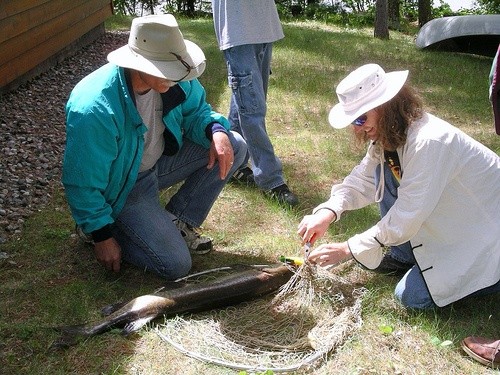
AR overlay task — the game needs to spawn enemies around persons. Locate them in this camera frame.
[212,0,298,210]
[61,14,247,281]
[297,63,500,312]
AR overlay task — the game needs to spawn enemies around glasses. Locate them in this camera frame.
[352,114,368,128]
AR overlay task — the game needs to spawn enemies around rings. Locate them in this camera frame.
[230,162,233,165]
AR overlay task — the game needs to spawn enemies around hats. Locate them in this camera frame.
[107,14,207,81]
[327,63,410,130]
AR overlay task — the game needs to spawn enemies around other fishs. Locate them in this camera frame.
[37,263,295,355]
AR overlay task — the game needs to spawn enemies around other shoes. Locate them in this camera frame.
[167,219,213,256]
[228,167,256,186]
[379,256,407,275]
[76,225,96,247]
[460,336,500,370]
[264,184,301,207]
